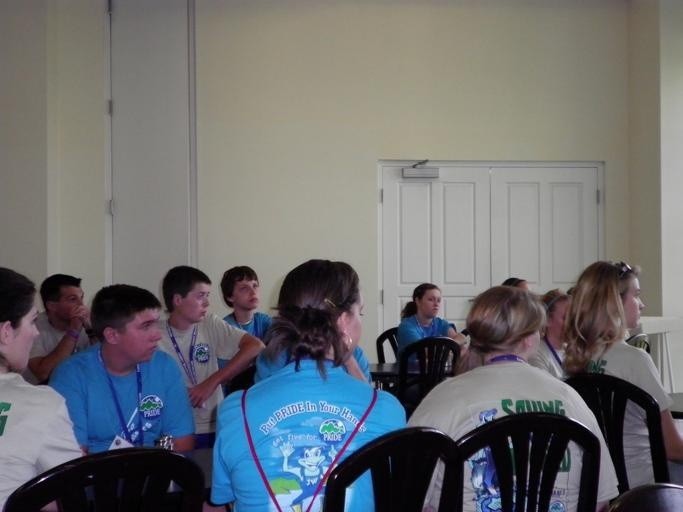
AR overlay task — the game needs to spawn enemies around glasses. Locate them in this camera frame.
[615,261,632,279]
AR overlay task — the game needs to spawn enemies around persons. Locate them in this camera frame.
[397,283,469,409]
[0,265,92,509]
[9,272,104,385]
[405,281,622,511]
[152,262,267,510]
[213,263,278,395]
[502,278,528,288]
[47,283,196,461]
[251,335,373,386]
[205,257,408,511]
[528,259,683,490]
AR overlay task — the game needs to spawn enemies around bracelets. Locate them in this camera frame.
[63,329,81,341]
[85,328,98,338]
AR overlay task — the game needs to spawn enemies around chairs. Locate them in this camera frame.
[320,425,465,511]
[224,366,257,400]
[607,483,680,510]
[1,447,207,510]
[561,371,672,504]
[391,335,462,424]
[446,412,604,509]
[376,326,403,367]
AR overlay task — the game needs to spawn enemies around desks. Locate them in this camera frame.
[367,362,456,421]
